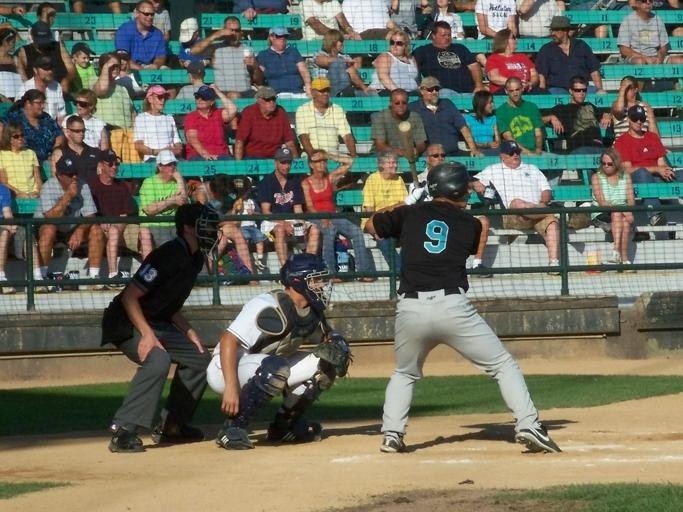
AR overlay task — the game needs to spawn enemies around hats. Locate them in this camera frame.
[542,15,577,28]
[29,20,95,70]
[144,76,329,99]
[627,105,645,119]
[54,148,179,175]
[185,61,204,75]
[498,140,520,154]
[273,147,293,163]
[268,25,290,37]
[178,17,198,42]
[419,76,440,88]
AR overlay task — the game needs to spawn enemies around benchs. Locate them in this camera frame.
[1,10,683,284]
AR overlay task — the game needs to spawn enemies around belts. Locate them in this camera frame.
[403,287,461,299]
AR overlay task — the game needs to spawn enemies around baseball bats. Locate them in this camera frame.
[399,121,419,188]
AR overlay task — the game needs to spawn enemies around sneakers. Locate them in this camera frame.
[622,261,636,273]
[514,421,562,453]
[547,260,560,276]
[0,271,126,294]
[150,423,204,444]
[608,249,620,263]
[108,430,144,452]
[215,427,253,450]
[379,432,406,452]
[470,264,493,278]
[649,213,666,226]
[264,422,321,443]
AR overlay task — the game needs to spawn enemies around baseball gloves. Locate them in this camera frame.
[312,329,351,377]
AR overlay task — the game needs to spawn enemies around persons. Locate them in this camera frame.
[365,161,561,454]
[205,252,351,450]
[0,2,683,294]
[100,203,223,454]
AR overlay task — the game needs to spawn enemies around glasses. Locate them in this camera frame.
[11,99,89,139]
[508,150,520,156]
[630,116,645,123]
[389,39,403,46]
[430,153,445,157]
[572,88,586,92]
[602,161,612,166]
[137,9,154,16]
[424,86,439,92]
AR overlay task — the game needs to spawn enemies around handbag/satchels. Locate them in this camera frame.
[566,212,591,230]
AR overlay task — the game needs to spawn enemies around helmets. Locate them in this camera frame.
[174,202,223,262]
[279,253,333,311]
[426,160,479,199]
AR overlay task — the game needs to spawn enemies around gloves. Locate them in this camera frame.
[403,182,425,206]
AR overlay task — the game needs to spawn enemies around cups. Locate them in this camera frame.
[68,271,79,290]
[52,271,63,293]
[668,222,676,240]
